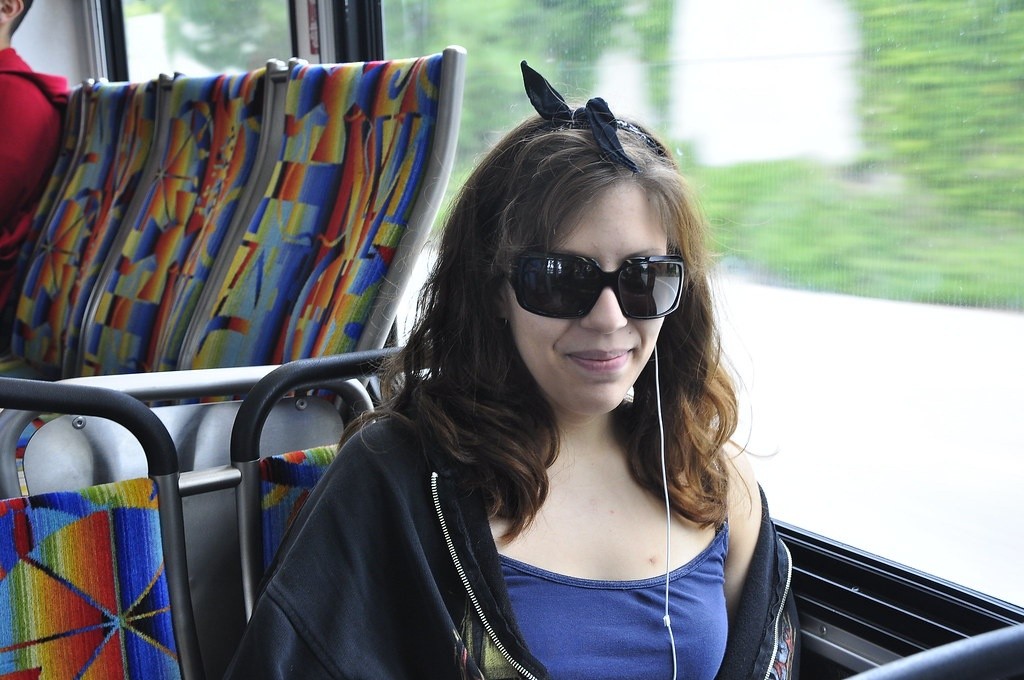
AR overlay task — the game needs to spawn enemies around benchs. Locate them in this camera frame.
[1,45,468,680]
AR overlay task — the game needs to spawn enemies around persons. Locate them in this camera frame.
[223,59,800,680]
[0,0,70,313]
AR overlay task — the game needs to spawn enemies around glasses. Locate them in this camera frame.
[502,250,686,319]
